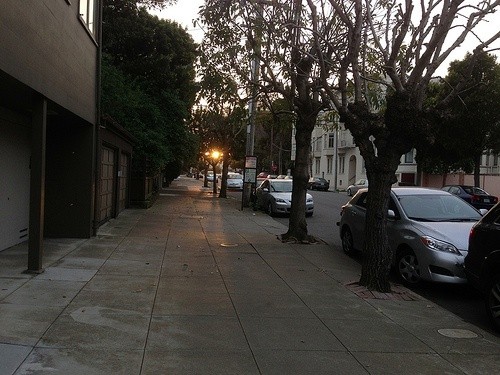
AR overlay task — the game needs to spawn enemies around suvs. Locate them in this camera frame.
[463,202,500,335]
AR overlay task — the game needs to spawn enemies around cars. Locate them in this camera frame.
[346,178,369,197]
[336,185,489,295]
[440,184,499,212]
[194,170,221,182]
[256,179,314,217]
[306,177,329,192]
[256,171,294,188]
[218,172,243,191]
